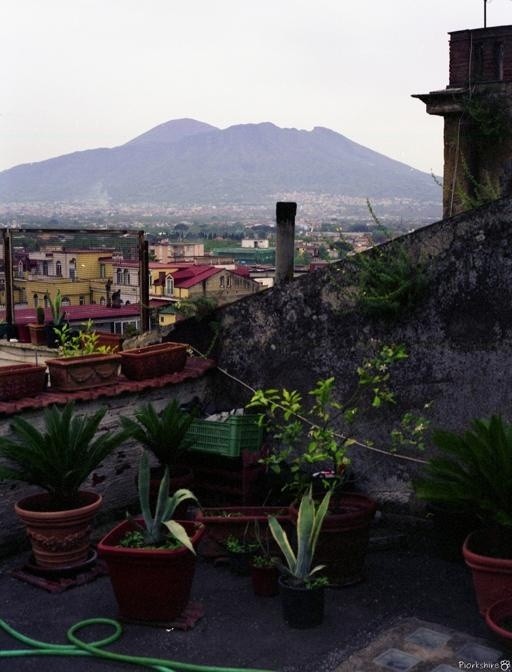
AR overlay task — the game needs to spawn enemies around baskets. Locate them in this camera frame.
[180,413,265,459]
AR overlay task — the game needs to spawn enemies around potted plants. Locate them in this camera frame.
[0,393,328,627]
[417,409,512,616]
[0,289,122,398]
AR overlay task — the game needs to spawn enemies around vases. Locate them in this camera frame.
[484,598,512,650]
[118,341,188,379]
[277,493,375,563]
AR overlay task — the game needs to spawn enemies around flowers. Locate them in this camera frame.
[245,340,430,512]
